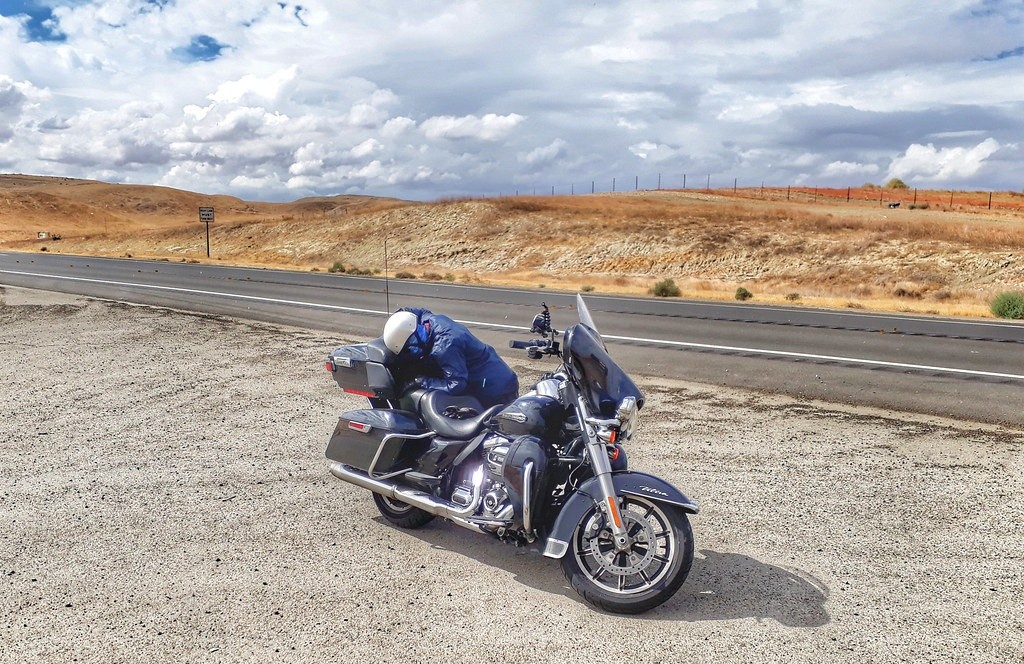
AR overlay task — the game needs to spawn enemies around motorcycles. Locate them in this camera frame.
[320,293,703,613]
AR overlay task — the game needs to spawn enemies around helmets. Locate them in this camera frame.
[384,311,417,355]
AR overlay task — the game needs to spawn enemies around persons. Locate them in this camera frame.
[385,306,520,410]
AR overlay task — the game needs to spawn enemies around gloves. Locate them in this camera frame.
[396,377,416,399]
